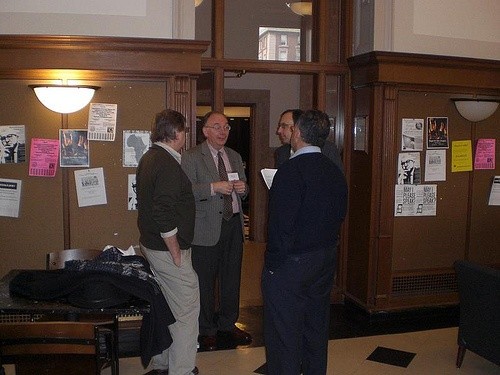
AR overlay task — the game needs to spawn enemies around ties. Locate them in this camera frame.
[217,150,234,224]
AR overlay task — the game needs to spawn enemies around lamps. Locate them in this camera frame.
[448,97,500,122]
[285,2,312,17]
[27,84,101,113]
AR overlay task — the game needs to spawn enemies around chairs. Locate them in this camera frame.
[46,248,115,375]
[0,316,101,375]
[453,258,500,369]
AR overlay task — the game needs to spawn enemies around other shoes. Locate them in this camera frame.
[143,365,199,375]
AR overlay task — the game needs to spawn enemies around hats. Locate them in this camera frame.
[73,279,128,309]
[24,269,63,300]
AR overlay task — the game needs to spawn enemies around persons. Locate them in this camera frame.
[136,109,201,375]
[181,111,252,351]
[275,109,345,176]
[260,109,348,375]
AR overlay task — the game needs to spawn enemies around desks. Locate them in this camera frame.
[0,269,151,375]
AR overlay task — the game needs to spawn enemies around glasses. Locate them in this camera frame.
[205,124,231,132]
[279,123,294,129]
[183,126,190,133]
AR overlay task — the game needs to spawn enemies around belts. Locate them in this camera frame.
[233,211,240,217]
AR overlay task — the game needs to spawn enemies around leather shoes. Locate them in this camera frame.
[217,323,252,345]
[197,329,238,352]
